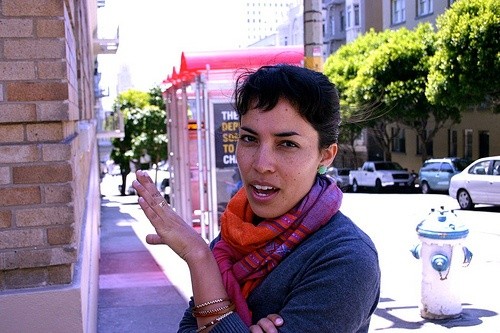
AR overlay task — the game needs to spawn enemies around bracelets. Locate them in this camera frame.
[189,296,237,333]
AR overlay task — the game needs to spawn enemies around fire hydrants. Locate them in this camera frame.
[409,205,473,322]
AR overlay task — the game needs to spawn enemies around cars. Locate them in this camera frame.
[448,156,500,210]
[323,167,353,194]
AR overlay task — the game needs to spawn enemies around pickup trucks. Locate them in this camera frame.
[348,161,412,192]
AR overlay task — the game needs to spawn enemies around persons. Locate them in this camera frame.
[119,148,150,196]
[132,61,382,333]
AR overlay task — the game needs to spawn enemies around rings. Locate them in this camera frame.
[159,200,166,208]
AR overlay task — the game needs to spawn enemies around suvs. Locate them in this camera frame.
[418,157,477,194]
